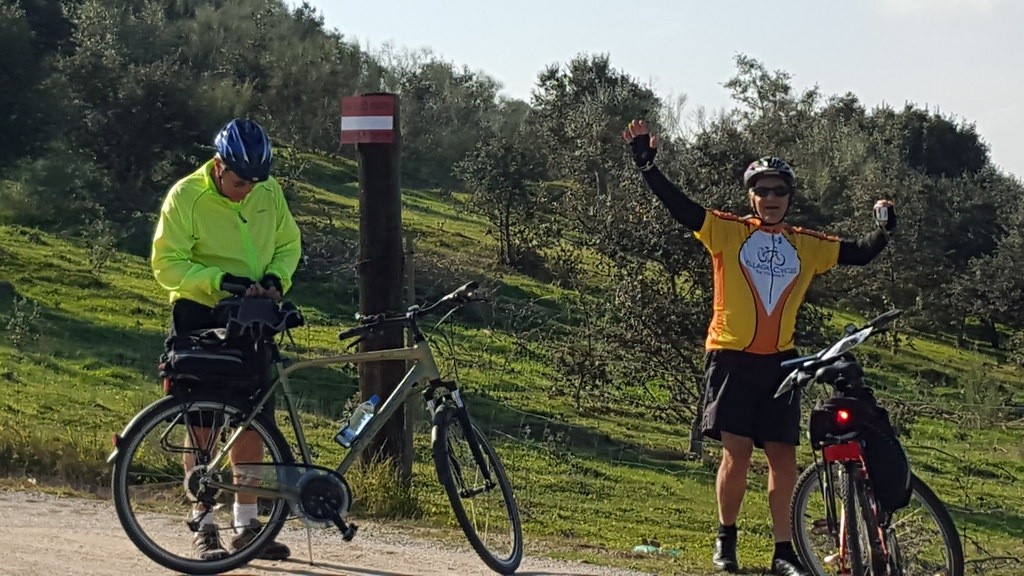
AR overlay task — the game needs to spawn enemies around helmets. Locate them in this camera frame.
[743,156,796,189]
[214,118,273,182]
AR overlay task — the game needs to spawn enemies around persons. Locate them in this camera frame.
[623,119,897,576]
[150,119,301,560]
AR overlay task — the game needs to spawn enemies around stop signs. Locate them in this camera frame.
[340,95,394,144]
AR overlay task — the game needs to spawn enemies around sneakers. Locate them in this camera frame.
[188,524,230,560]
[231,518,291,560]
[770,554,813,576]
[712,533,738,572]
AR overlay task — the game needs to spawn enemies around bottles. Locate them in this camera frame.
[335,395,380,448]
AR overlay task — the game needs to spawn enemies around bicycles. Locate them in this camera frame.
[780,308,964,576]
[107,280,523,576]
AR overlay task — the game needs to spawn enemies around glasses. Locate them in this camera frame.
[751,187,792,197]
